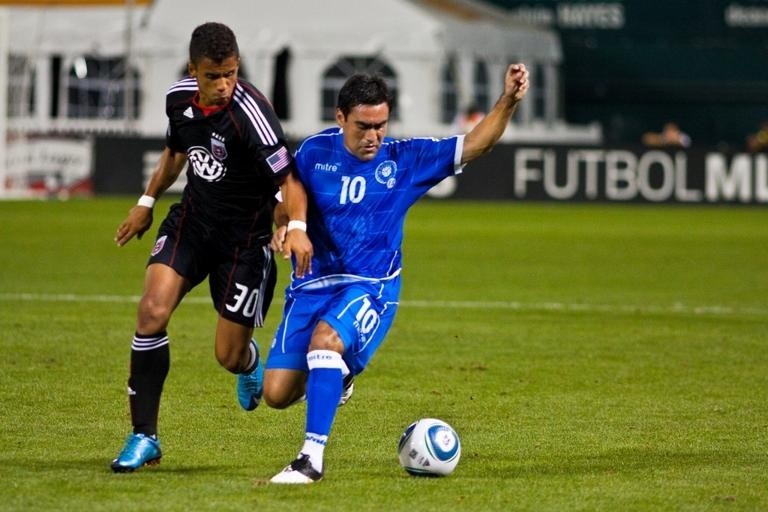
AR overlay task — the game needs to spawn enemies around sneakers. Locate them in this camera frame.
[270,455,324,485]
[236,337,264,411]
[110,432,162,473]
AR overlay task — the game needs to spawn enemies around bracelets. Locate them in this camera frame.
[136,194,157,208]
[287,220,307,232]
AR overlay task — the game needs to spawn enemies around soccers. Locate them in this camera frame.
[396,419,461,476]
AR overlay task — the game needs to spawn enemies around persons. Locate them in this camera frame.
[263,62,529,483]
[110,22,314,473]
